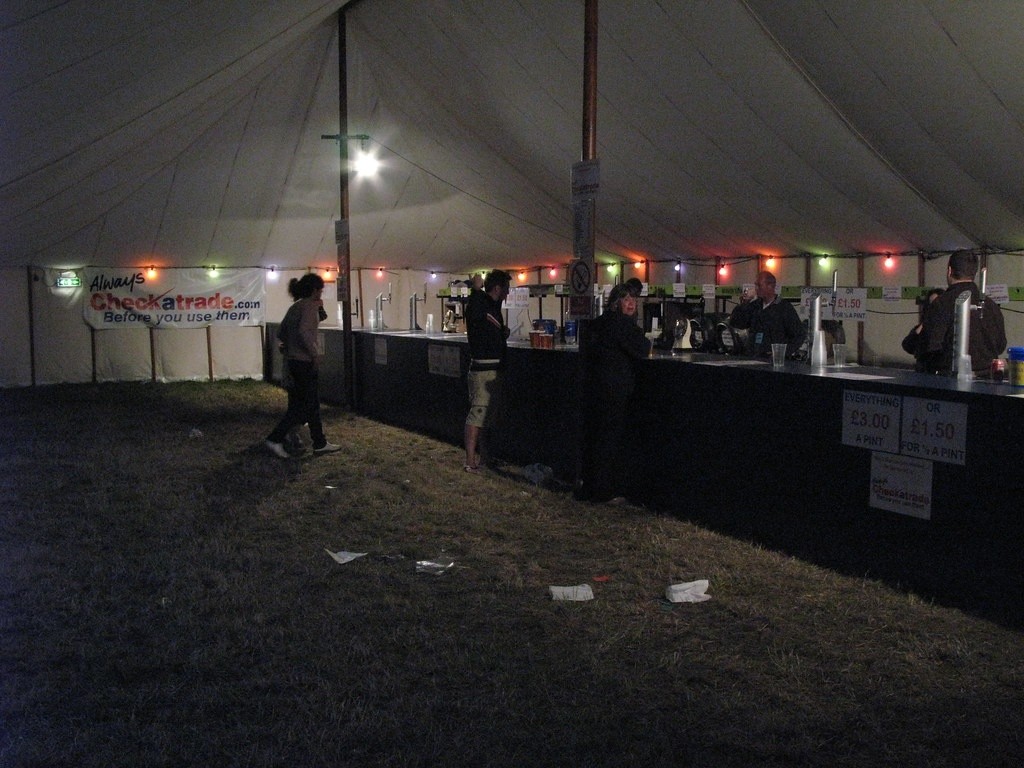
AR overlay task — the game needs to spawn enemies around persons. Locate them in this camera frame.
[730,272,806,356]
[584,278,651,505]
[264,273,341,459]
[463,269,512,473]
[902,250,1008,378]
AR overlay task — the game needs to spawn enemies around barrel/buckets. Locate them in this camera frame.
[533,319,555,335]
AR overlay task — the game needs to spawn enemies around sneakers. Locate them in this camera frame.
[313,441,342,455]
[264,438,290,459]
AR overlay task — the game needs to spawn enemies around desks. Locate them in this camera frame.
[265,321,1024,636]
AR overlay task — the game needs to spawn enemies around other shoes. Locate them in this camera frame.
[284,432,307,452]
[463,459,478,473]
[608,497,625,505]
[490,455,509,473]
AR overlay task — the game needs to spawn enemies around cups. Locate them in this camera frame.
[957,355,972,380]
[811,331,827,365]
[369,310,376,329]
[832,344,846,366]
[539,334,552,348]
[771,344,787,366]
[426,314,434,334]
[529,331,544,346]
